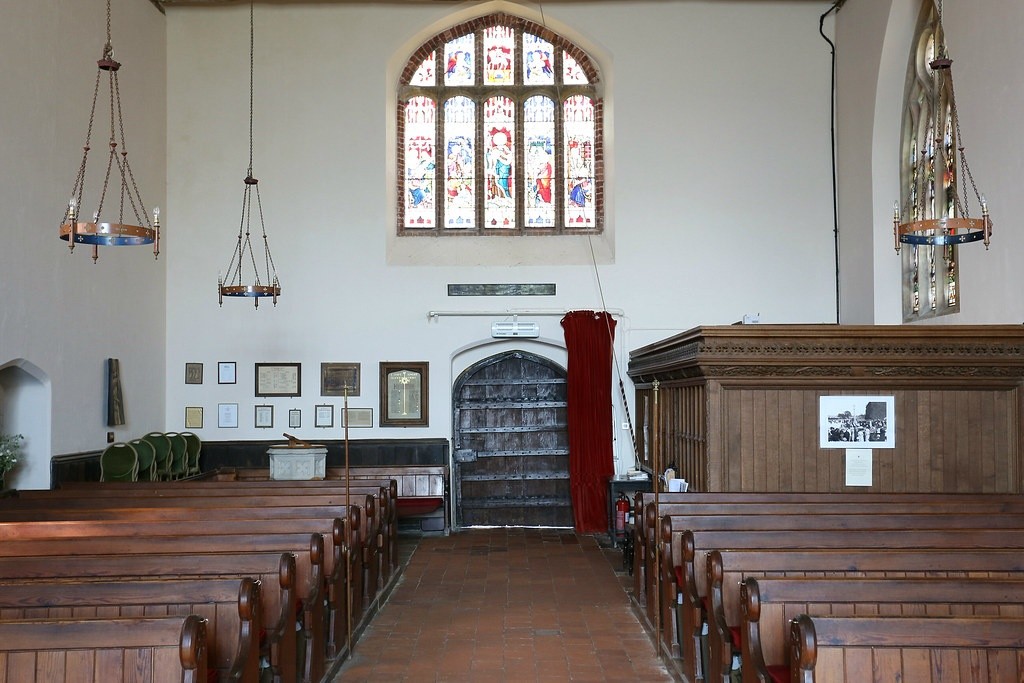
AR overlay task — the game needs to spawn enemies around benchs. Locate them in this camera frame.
[0,466,451,683]
[634,489,1024,683]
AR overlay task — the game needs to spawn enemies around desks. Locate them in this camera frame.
[606,477,653,548]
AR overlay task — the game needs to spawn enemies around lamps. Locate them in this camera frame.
[217,0,281,310]
[60,0,160,265]
[893,0,992,264]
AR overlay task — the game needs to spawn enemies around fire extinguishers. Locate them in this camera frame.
[613,490,631,538]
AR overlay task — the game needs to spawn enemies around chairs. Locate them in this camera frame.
[100,431,202,482]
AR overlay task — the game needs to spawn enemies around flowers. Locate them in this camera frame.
[0,433,25,488]
